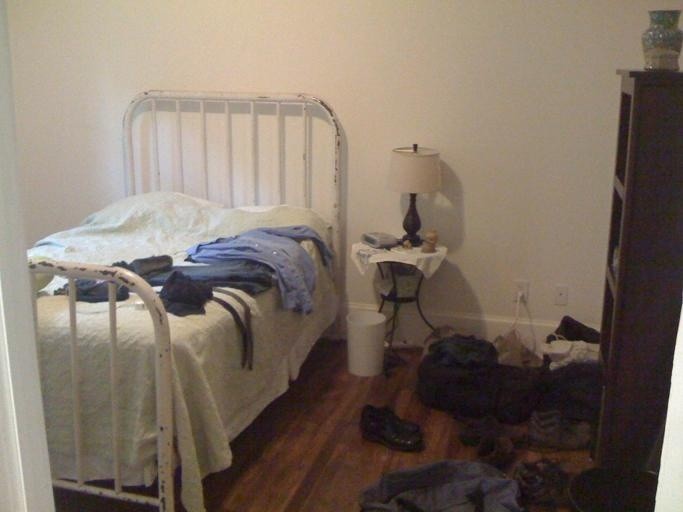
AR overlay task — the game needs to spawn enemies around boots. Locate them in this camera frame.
[528,409,593,453]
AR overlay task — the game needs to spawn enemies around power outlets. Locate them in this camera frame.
[512,279,529,302]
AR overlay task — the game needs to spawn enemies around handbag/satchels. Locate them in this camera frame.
[357,457,525,512]
[412,331,500,420]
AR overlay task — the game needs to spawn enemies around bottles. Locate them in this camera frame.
[639,8,683,72]
[420,228,438,253]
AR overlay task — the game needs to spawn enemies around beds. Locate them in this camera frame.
[24,90,342,512]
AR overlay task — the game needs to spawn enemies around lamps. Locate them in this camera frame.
[387,144,443,252]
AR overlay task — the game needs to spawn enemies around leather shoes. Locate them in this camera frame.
[363,419,426,454]
[358,403,421,435]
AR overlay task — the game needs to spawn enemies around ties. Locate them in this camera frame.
[211,285,254,371]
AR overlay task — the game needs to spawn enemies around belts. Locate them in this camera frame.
[209,295,247,369]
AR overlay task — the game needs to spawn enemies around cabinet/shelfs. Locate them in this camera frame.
[594,68,683,472]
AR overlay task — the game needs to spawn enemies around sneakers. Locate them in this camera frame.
[512,463,569,506]
[459,416,522,448]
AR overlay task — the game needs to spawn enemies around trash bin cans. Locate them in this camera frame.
[346,310,386,377]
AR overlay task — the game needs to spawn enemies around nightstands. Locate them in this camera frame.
[351,243,447,361]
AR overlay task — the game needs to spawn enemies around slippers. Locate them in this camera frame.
[468,435,518,469]
